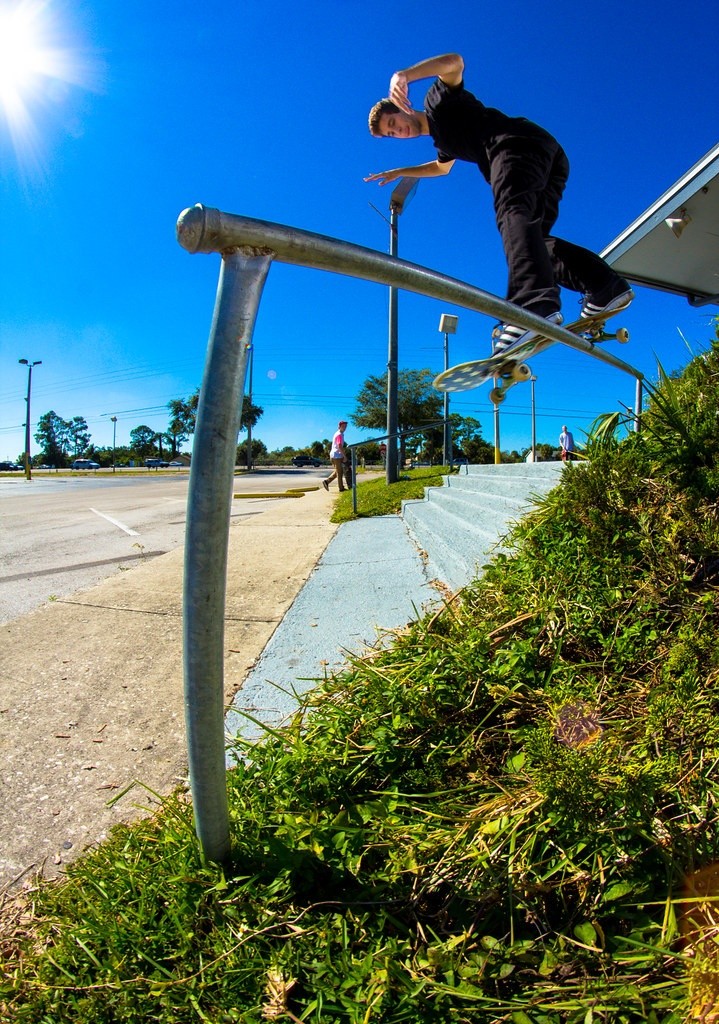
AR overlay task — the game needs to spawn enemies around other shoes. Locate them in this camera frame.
[322,480,329,491]
[339,489,347,492]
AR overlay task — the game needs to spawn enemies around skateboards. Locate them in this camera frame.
[432,298,633,404]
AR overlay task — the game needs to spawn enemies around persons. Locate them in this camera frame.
[322,421,348,492]
[343,441,352,490]
[559,426,573,460]
[363,53,635,361]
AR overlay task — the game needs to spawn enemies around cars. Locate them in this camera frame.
[169,461,184,466]
[73,460,100,470]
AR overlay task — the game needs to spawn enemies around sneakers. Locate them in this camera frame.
[577,278,635,320]
[490,304,563,359]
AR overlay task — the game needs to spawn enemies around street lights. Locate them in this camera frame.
[387,174,420,482]
[111,415,118,473]
[439,314,459,466]
[531,375,538,462]
[490,329,502,463]
[18,358,42,474]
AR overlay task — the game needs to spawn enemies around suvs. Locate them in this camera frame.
[144,458,169,468]
[292,455,323,468]
